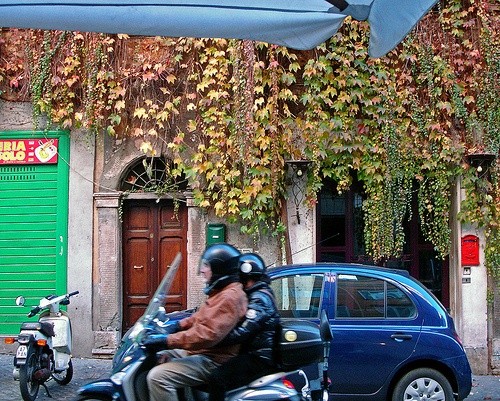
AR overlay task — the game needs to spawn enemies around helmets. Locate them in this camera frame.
[237,252,268,274]
[201,243,240,295]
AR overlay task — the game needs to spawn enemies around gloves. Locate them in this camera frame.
[143,334,168,354]
[155,320,180,334]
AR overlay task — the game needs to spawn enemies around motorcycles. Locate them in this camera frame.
[75,252,324,401]
[5,291,79,400]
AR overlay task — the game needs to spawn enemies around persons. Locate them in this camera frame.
[140,243,248,401]
[210,254,279,401]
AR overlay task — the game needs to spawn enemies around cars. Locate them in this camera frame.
[111,263,472,401]
